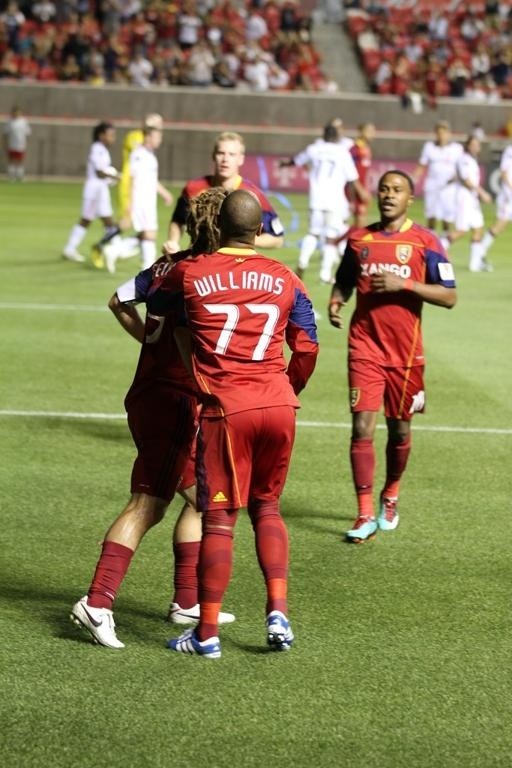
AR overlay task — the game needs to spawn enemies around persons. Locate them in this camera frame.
[411,119,511,273]
[68,188,237,650]
[328,170,456,543]
[63,112,174,275]
[2,108,33,180]
[279,116,377,283]
[149,194,318,659]
[162,131,285,256]
[1,1,512,115]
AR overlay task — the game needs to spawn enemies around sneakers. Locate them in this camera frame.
[165,627,223,660]
[168,602,236,627]
[66,236,192,274]
[344,517,379,544]
[68,593,124,650]
[377,506,399,532]
[266,611,296,651]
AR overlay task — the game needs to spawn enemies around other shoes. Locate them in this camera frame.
[483,259,493,274]
[319,272,335,281]
[295,261,307,280]
[468,262,480,272]
